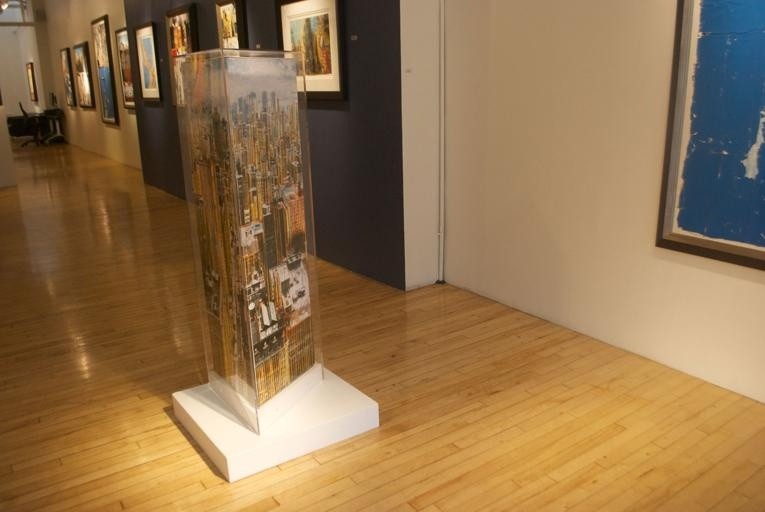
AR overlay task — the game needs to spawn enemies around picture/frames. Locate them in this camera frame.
[58,0,349,126]
[25,62,39,102]
[655,2,764,273]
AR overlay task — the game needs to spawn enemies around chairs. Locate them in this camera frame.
[18,102,46,146]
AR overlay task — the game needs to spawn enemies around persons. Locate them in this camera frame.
[221,8,236,37]
[301,18,317,76]
[313,16,332,75]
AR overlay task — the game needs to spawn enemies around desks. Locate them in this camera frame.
[43,109,66,145]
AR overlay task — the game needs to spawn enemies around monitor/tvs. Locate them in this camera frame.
[48,92,56,106]
